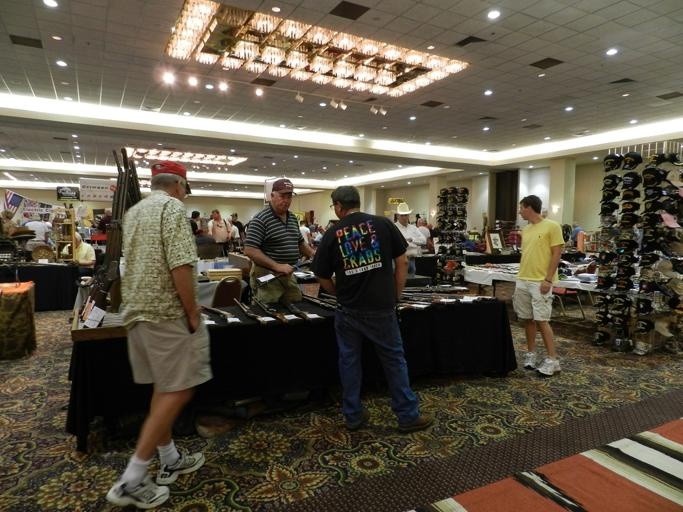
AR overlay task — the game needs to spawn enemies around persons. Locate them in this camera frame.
[393,202,426,275]
[513,196,562,376]
[571,223,584,245]
[417,218,429,249]
[190,210,244,256]
[310,187,433,432]
[1,208,112,269]
[244,179,315,304]
[106,161,212,509]
[300,218,323,247]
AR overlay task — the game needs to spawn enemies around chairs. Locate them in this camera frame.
[210,276,242,306]
[552,261,598,320]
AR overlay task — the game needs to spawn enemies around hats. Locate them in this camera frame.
[590,152,683,356]
[394,202,413,214]
[152,160,191,194]
[272,179,296,195]
[434,187,469,285]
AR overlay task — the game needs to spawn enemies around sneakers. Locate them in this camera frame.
[105,475,169,509]
[399,416,432,432]
[538,358,561,376]
[524,352,536,369]
[347,410,369,429]
[156,450,205,484]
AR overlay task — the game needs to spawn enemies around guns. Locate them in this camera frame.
[201,305,240,325]
[256,258,313,287]
[234,298,271,325]
[255,297,292,324]
[78,148,142,328]
[288,304,313,322]
[397,287,470,311]
[302,293,337,310]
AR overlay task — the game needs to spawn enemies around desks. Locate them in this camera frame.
[0,263,94,311]
[64,292,518,452]
[0,279,37,361]
[462,261,653,320]
